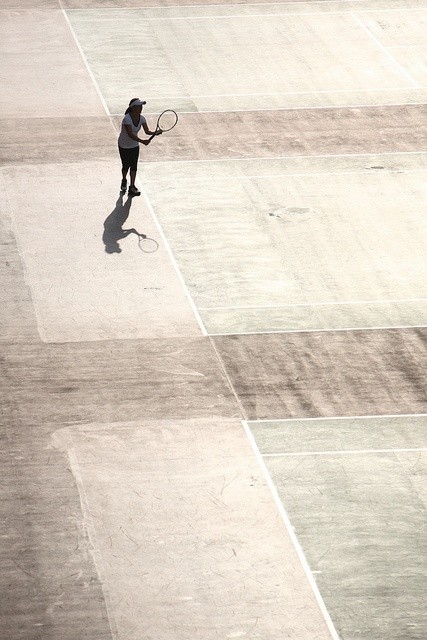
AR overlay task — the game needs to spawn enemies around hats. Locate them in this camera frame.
[129,99,147,108]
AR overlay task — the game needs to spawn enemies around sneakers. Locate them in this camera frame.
[128,185,141,195]
[121,178,127,191]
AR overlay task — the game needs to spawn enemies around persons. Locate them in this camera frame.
[118,98,162,195]
